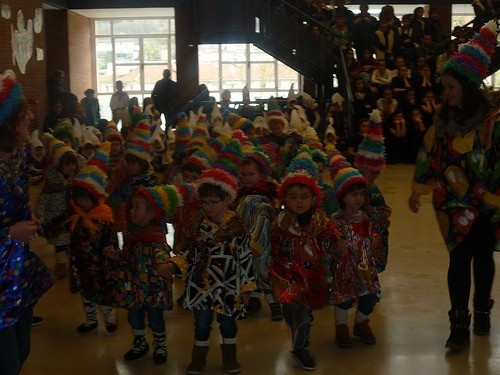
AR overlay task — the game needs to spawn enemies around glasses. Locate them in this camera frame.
[197,199,221,208]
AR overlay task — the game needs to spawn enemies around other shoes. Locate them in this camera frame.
[103,308,118,333]
[293,349,318,371]
[248,297,262,312]
[31,315,43,326]
[269,302,283,320]
[76,320,98,334]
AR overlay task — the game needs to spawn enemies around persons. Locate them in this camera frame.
[408,18,500,349]
[0,0,470,375]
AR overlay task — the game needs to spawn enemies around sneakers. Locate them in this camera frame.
[335,323,352,349]
[353,319,376,345]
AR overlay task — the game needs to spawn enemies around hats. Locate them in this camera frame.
[27,88,386,224]
[0,68,32,125]
[442,19,498,88]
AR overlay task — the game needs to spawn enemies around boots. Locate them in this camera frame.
[124,334,149,361]
[152,333,167,365]
[219,338,241,373]
[186,340,210,375]
[472,298,494,336]
[445,310,471,349]
